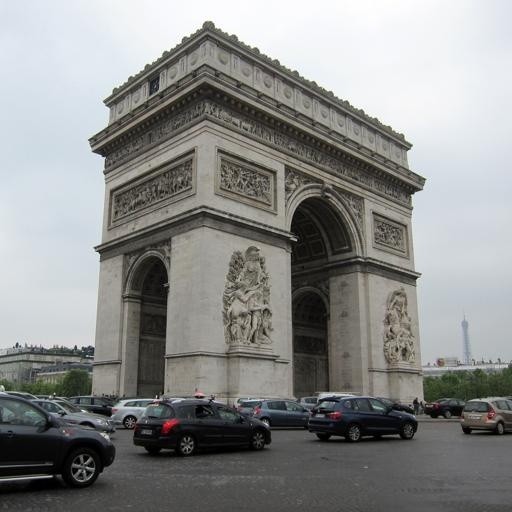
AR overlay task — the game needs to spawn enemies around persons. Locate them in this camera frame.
[411,396,421,416]
[228,246,273,346]
[383,287,418,363]
